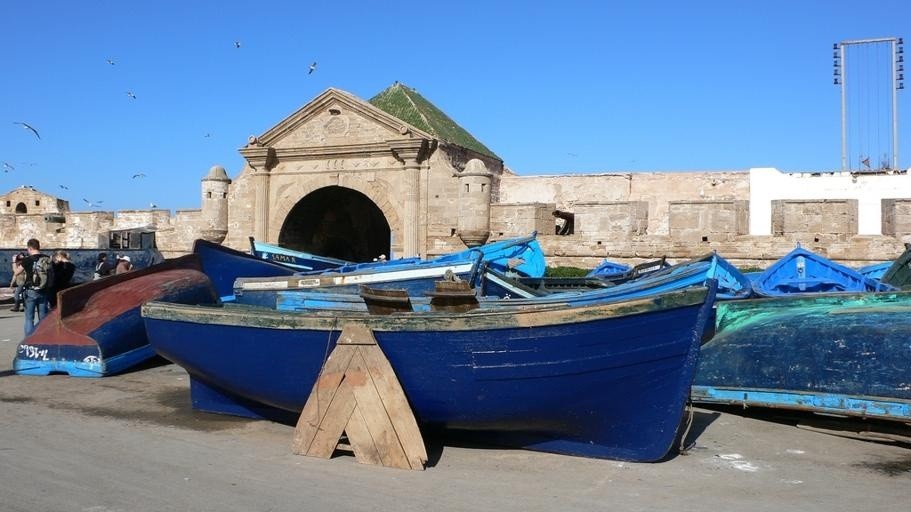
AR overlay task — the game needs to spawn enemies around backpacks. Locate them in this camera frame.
[27,257,56,291]
[92,261,104,280]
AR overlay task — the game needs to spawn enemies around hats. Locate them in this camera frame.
[119,255,131,262]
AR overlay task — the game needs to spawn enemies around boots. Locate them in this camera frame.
[10,301,20,311]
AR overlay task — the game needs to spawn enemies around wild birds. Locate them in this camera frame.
[309,61,317,75]
[12,121,41,140]
[1,160,15,174]
[82,196,105,208]
[233,39,241,49]
[150,202,157,208]
[106,58,115,66]
[60,183,69,192]
[131,171,146,179]
[124,91,137,101]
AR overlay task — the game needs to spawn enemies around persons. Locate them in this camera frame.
[369,254,387,263]
[10,252,27,312]
[11,239,51,337]
[115,256,133,274]
[47,250,76,311]
[94,253,116,279]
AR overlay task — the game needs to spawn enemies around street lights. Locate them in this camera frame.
[833,38,904,171]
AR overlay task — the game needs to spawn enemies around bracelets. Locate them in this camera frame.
[12,261,16,263]
